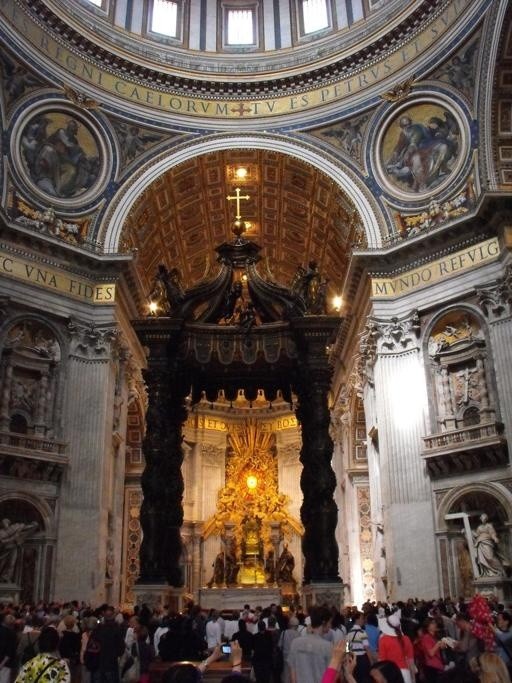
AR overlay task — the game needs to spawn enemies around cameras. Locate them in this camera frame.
[222,645,232,654]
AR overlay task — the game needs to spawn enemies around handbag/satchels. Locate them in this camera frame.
[118,648,141,682]
[441,643,455,665]
[84,640,101,670]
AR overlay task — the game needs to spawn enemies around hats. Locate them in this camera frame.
[289,616,299,625]
[379,616,404,636]
[122,608,131,616]
[64,615,76,625]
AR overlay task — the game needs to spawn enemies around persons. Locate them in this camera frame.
[221,280,246,320]
[338,120,361,159]
[469,512,507,577]
[422,116,450,140]
[297,260,323,297]
[154,262,185,305]
[0,588,512,682]
[1,517,28,553]
[207,551,225,585]
[277,546,296,581]
[446,54,471,90]
[384,112,453,189]
[115,122,149,162]
[46,118,94,198]
[5,66,31,99]
[222,549,242,586]
[21,119,49,169]
[265,550,278,584]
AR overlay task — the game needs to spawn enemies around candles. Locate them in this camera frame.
[274,551,275,568]
[224,551,225,567]
[255,554,256,563]
[265,558,266,568]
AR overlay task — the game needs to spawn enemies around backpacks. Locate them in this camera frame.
[21,632,39,665]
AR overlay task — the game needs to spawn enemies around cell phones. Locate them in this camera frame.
[345,639,350,654]
